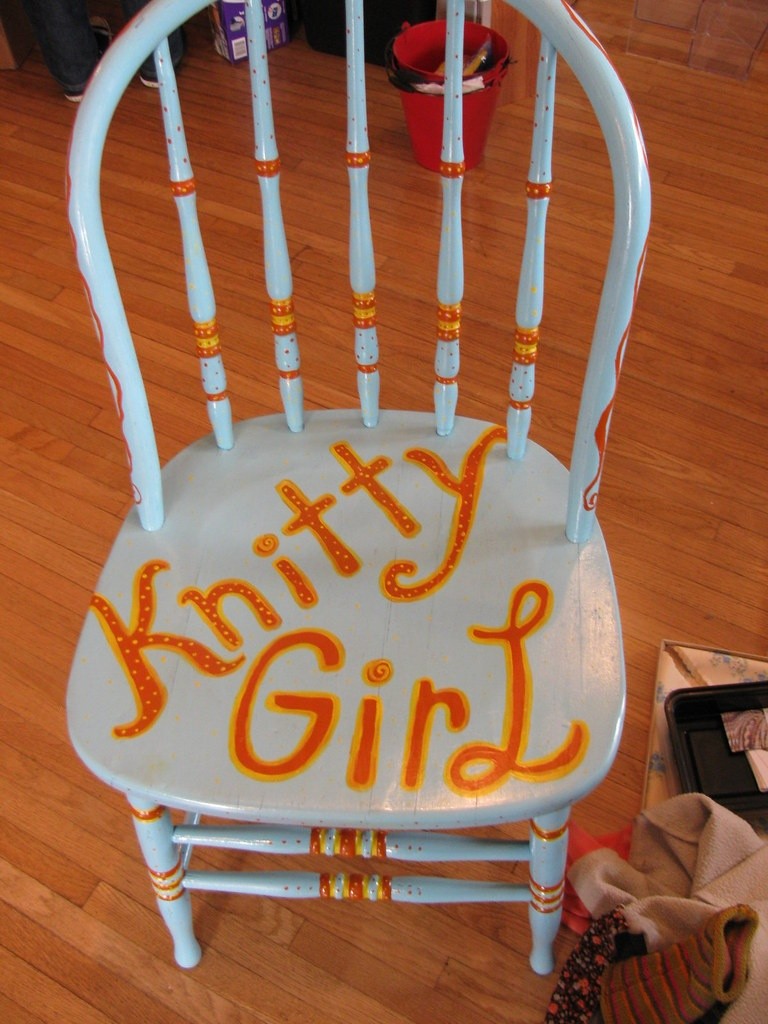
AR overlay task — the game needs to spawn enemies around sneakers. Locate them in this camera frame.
[64,16,112,102]
[139,24,186,88]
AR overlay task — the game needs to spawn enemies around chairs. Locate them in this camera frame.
[65,0,661,983]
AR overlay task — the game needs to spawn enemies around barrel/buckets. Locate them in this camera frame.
[385,19,511,173]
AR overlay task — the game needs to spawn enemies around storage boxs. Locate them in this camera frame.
[200,0,290,66]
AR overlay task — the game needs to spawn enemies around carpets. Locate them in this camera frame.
[619,636,768,1021]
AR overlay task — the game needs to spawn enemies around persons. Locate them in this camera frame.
[22,0,185,102]
